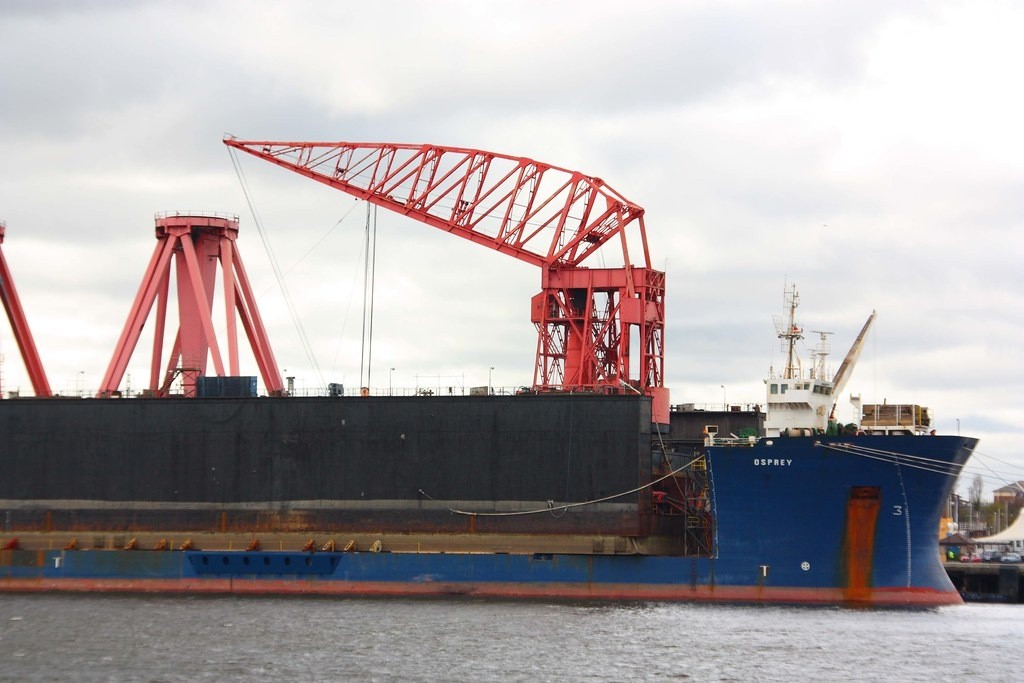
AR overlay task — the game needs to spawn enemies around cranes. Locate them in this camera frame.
[220,126,669,453]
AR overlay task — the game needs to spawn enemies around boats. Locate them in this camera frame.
[0,276,975,613]
